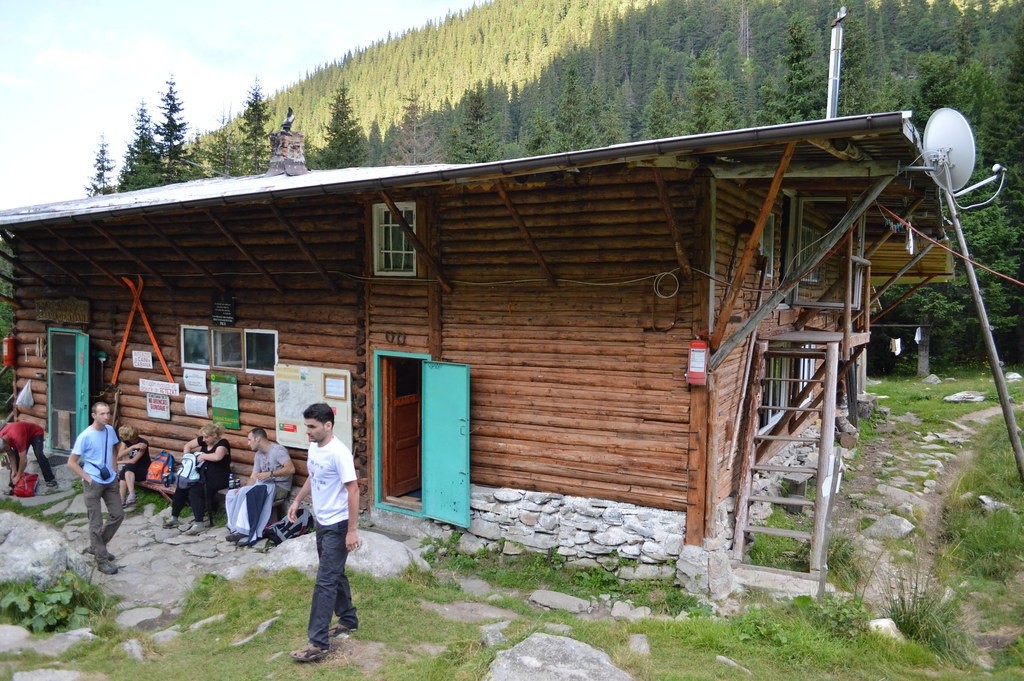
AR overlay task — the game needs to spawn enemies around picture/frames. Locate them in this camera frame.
[323,373,347,400]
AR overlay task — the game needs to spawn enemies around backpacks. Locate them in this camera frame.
[264,505,314,547]
[146,453,175,486]
[176,451,206,490]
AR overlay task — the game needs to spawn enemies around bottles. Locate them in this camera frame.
[228,473,234,489]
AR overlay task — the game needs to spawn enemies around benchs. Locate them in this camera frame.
[131,478,283,528]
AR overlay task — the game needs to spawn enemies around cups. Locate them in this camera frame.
[162,473,170,487]
[235,479,240,488]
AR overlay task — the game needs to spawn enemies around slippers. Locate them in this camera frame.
[46,481,57,487]
[8,489,14,495]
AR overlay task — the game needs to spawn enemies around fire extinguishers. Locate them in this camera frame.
[1,332,16,367]
[684,335,709,392]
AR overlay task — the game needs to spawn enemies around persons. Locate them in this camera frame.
[118,424,151,506]
[226,428,296,545]
[0,422,58,495]
[165,424,232,535]
[288,402,360,660]
[68,402,124,574]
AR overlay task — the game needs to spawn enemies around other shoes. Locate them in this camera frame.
[122,491,135,506]
[98,562,119,575]
[291,643,327,661]
[329,622,347,637]
[163,519,181,529]
[186,520,205,535]
[87,545,116,562]
[227,532,244,541]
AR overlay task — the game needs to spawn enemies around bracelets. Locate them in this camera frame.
[270,471,275,478]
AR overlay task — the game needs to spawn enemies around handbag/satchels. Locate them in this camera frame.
[100,467,110,481]
[16,378,34,407]
[13,472,38,495]
